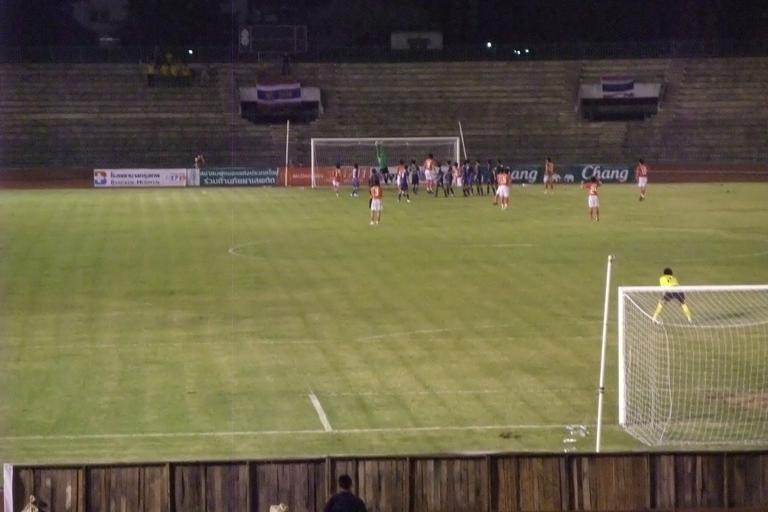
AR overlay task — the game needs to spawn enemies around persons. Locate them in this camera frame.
[543,158,554,193]
[331,140,512,225]
[195,154,205,170]
[653,268,692,325]
[146,51,193,88]
[580,177,603,222]
[636,160,647,201]
[281,50,290,75]
